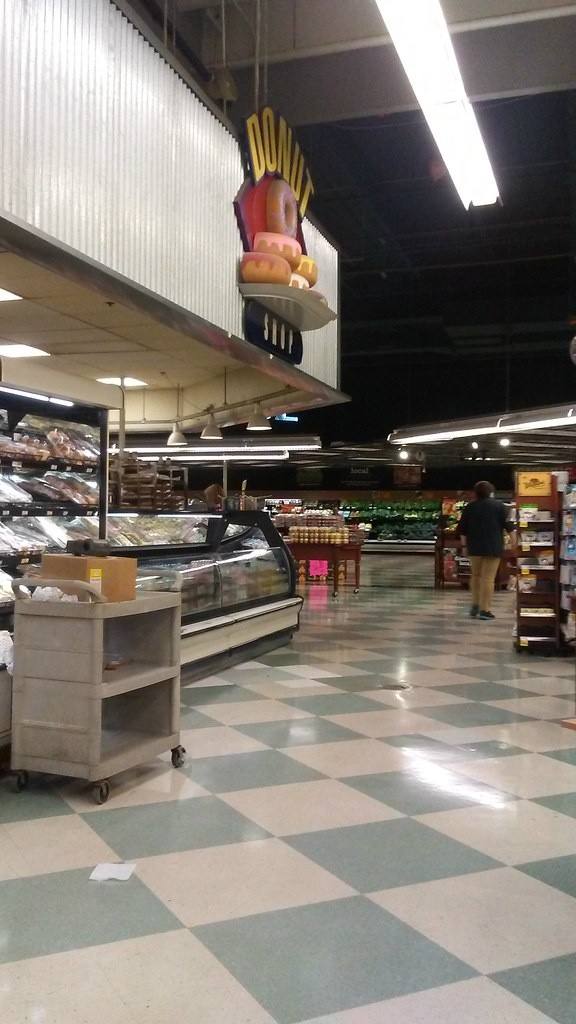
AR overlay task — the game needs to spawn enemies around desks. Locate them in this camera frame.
[289,543,361,597]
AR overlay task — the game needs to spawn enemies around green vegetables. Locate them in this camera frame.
[339,498,443,541]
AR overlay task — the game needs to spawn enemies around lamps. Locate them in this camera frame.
[457,452,468,462]
[202,65,239,102]
[482,450,487,461]
[471,452,478,461]
[386,404,576,446]
[247,403,272,431]
[167,421,188,446]
[415,450,425,462]
[375,1,502,210]
[200,414,223,440]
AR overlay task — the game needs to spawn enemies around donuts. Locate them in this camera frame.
[242,179,330,307]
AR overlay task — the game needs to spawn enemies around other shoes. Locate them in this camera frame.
[470,606,478,617]
[479,611,496,620]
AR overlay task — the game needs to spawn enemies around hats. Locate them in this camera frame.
[475,481,495,498]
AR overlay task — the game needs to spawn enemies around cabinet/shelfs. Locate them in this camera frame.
[437,471,576,657]
[0,388,110,659]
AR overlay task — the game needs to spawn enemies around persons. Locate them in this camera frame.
[457,482,515,619]
